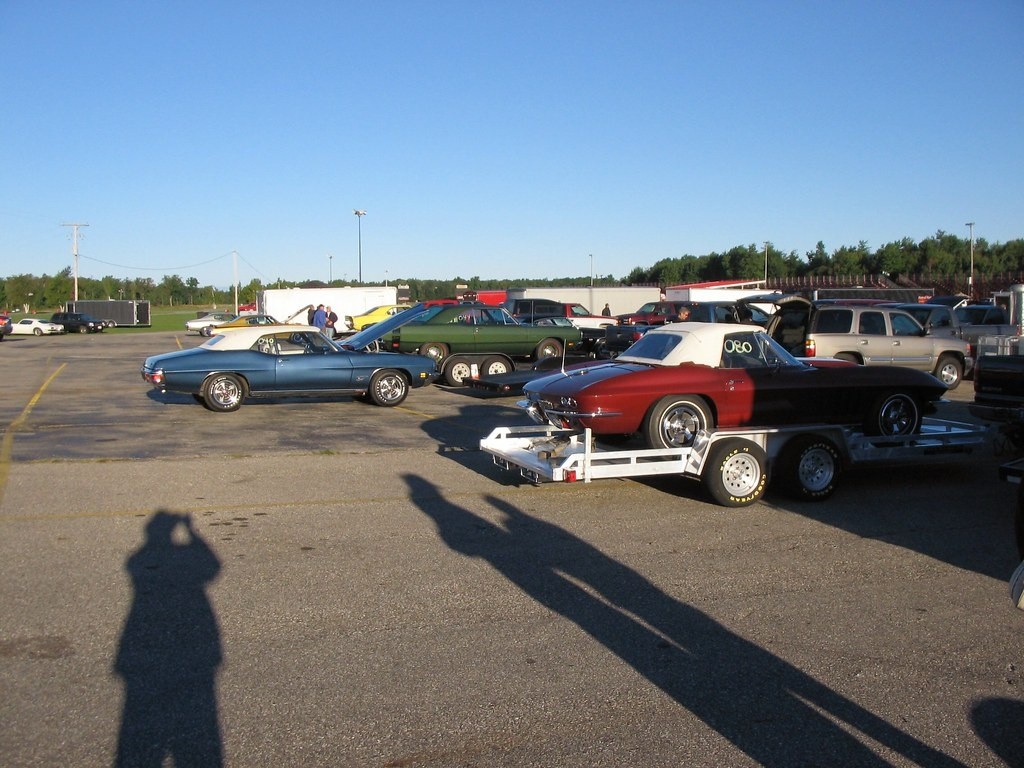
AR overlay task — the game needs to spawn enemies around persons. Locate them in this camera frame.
[602,303,611,316]
[674,305,692,323]
[308,304,338,339]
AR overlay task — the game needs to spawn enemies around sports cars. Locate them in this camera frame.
[516,320,947,460]
[143,304,441,408]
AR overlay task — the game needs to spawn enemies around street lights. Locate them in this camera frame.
[353,208,367,285]
[965,222,975,298]
[386,270,389,287]
[330,255,335,283]
[589,254,594,287]
[763,240,770,283]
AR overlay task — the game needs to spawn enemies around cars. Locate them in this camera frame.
[345,304,413,332]
[0,316,12,341]
[184,312,240,337]
[206,304,312,345]
[9,318,65,336]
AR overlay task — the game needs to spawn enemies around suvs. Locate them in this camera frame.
[737,294,974,393]
[417,284,1024,393]
[394,303,584,366]
[50,311,104,334]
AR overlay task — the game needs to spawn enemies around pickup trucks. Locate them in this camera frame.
[966,354,1024,450]
